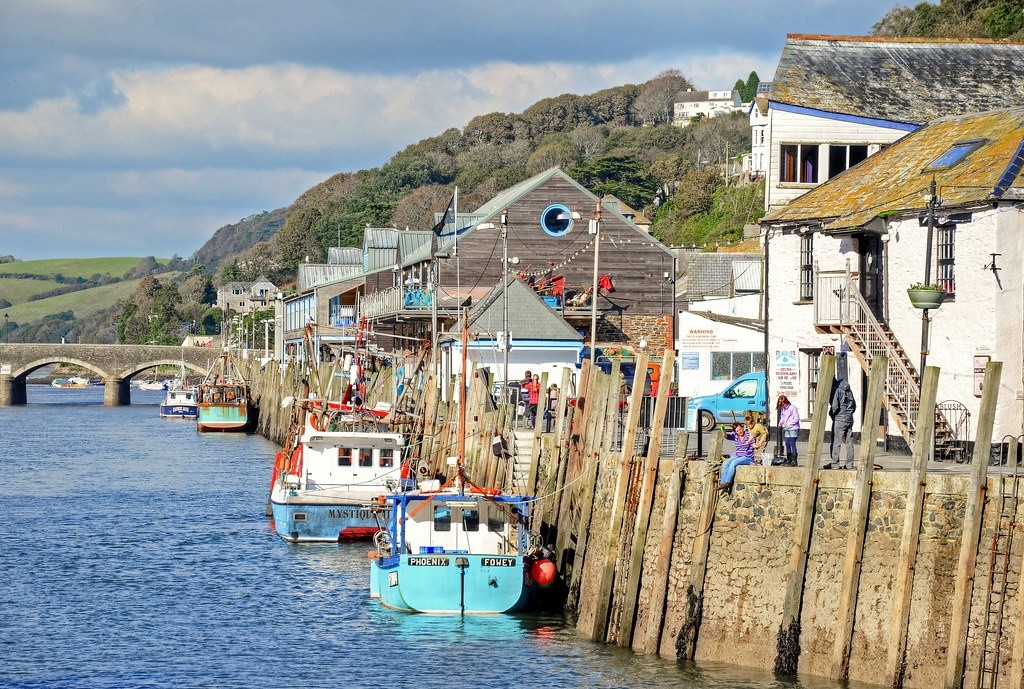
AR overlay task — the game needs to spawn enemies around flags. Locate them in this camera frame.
[432,191,455,237]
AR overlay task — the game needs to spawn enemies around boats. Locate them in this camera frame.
[159,350,199,420]
[266,313,408,539]
[365,306,533,615]
[194,318,251,434]
[139,382,165,390]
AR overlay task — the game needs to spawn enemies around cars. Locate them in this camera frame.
[687,371,766,431]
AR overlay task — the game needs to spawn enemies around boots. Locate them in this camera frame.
[781,453,797,467]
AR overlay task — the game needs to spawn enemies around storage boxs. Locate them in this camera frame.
[543,298,557,307]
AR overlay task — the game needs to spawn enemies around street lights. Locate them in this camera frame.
[4,312,10,342]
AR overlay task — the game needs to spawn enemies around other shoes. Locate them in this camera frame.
[824,463,839,469]
[840,465,853,469]
[716,483,729,490]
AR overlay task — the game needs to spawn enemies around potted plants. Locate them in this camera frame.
[907,282,948,309]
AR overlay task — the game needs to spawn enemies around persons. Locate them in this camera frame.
[822,377,856,470]
[194,341,211,348]
[716,410,769,496]
[519,371,559,431]
[776,396,800,467]
